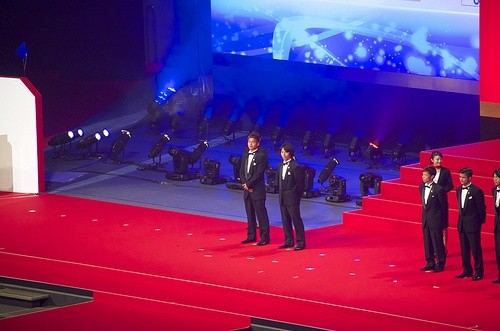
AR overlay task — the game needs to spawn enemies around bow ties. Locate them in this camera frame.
[461,184,472,189]
[496,189,500,192]
[425,183,433,188]
[248,150,258,155]
[283,161,291,165]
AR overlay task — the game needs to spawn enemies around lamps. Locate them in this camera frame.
[48,85,407,204]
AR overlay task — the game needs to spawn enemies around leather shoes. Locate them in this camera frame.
[473,276,481,279]
[242,239,254,243]
[456,272,472,277]
[494,279,500,283]
[257,240,266,245]
[420,266,433,270]
[295,247,303,250]
[434,266,442,272]
[280,244,293,248]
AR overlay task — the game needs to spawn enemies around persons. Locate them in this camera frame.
[278,142,305,250]
[418,166,449,273]
[455,167,486,281]
[239,132,270,246]
[419,151,454,259]
[491,169,500,283]
